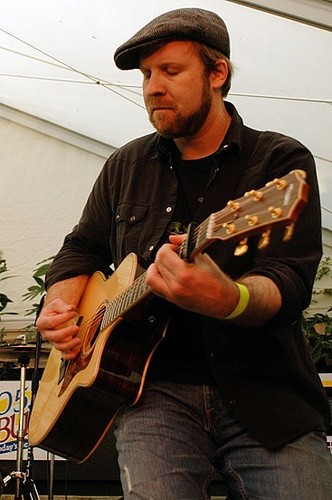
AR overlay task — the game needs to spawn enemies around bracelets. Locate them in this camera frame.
[223,283,249,321]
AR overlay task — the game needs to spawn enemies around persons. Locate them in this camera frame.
[33,9,332,500]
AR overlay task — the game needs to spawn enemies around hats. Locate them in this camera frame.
[115,4,231,69]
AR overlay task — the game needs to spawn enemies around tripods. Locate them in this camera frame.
[3,355,41,500]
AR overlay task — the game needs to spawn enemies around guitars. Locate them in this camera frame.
[30,169,310,465]
